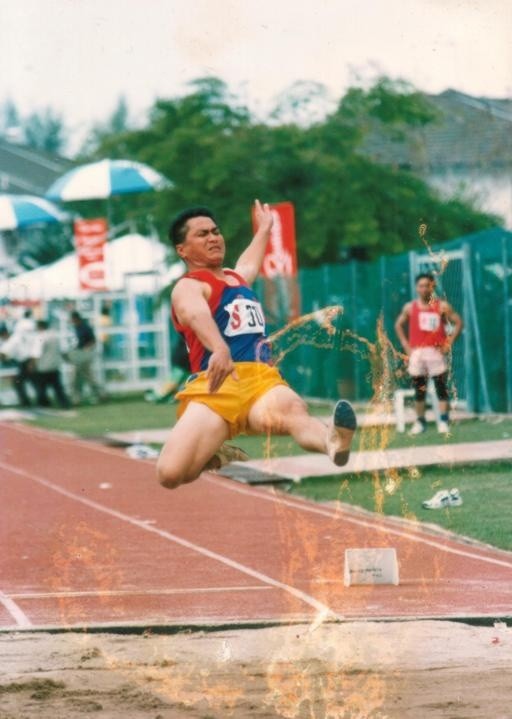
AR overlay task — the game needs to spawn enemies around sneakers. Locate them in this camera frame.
[421,487,463,510]
[409,416,450,437]
[213,444,250,471]
[325,399,357,467]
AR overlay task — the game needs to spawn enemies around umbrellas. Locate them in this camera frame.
[0,158,176,242]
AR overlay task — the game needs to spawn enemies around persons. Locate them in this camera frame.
[2,309,105,407]
[156,200,358,490]
[395,274,463,437]
